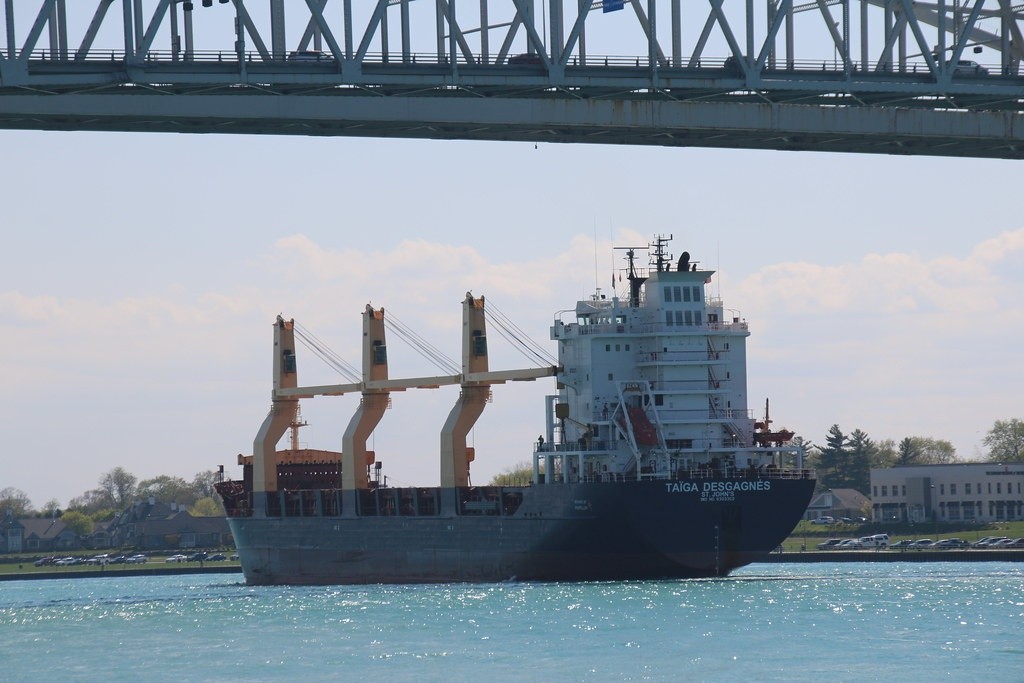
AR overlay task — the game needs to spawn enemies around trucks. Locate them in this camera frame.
[858,534,890,550]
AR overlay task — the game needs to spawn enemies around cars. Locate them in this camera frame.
[906,539,933,550]
[34,556,86,566]
[205,554,227,562]
[508,52,552,66]
[109,555,127,565]
[932,535,1024,550]
[287,49,334,63]
[818,539,842,550]
[186,553,208,562]
[723,56,765,76]
[855,516,869,523]
[889,539,914,550]
[229,554,238,562]
[125,554,148,565]
[166,554,188,564]
[833,539,856,551]
[839,517,853,525]
[943,59,991,80]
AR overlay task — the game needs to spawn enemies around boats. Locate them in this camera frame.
[212,229,820,589]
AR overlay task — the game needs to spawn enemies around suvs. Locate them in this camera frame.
[810,516,836,525]
[86,554,109,566]
